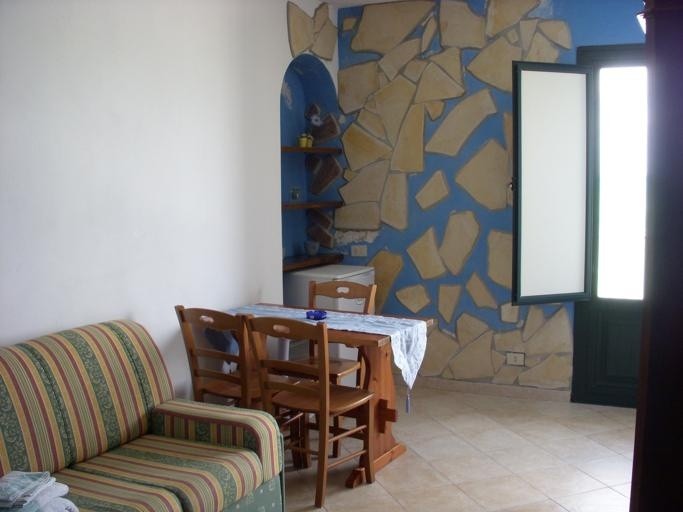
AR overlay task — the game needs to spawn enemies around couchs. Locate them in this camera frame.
[0,319,284,511]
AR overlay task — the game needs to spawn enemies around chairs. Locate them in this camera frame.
[260,281,377,457]
[242,313,376,508]
[175,305,302,471]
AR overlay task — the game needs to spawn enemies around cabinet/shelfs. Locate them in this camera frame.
[281,103,344,273]
[284,264,376,418]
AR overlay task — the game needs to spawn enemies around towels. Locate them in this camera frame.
[0,470,77,511]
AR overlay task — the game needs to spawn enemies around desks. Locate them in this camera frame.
[203,302,433,489]
